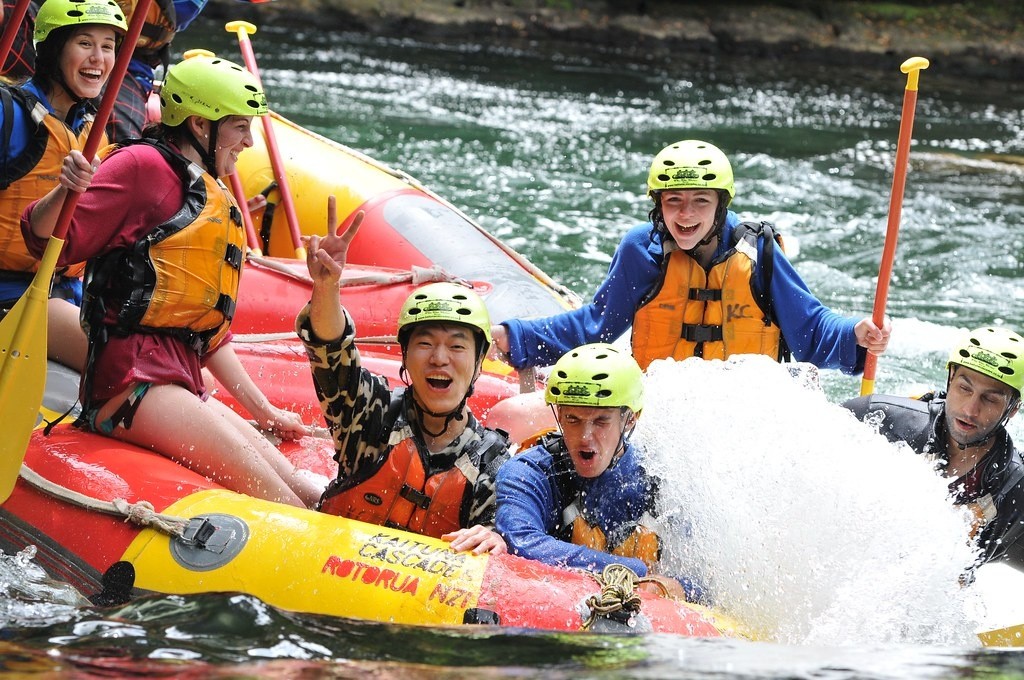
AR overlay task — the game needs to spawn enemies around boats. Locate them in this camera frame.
[0,53,1024,651]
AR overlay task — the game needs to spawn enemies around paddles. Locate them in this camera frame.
[860,56,932,396]
[225,20,319,262]
[0,0,151,505]
[184,48,270,259]
[1,1,32,75]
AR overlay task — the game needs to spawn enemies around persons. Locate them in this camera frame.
[842,326,1024,574]
[488,140,892,374]
[18,54,323,509]
[495,342,715,606]
[295,195,512,556]
[0,0,208,374]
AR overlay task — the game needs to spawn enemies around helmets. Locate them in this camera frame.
[544,342,646,413]
[946,326,1024,397]
[397,282,491,351]
[33,0,128,44]
[159,58,269,126]
[648,140,735,200]
